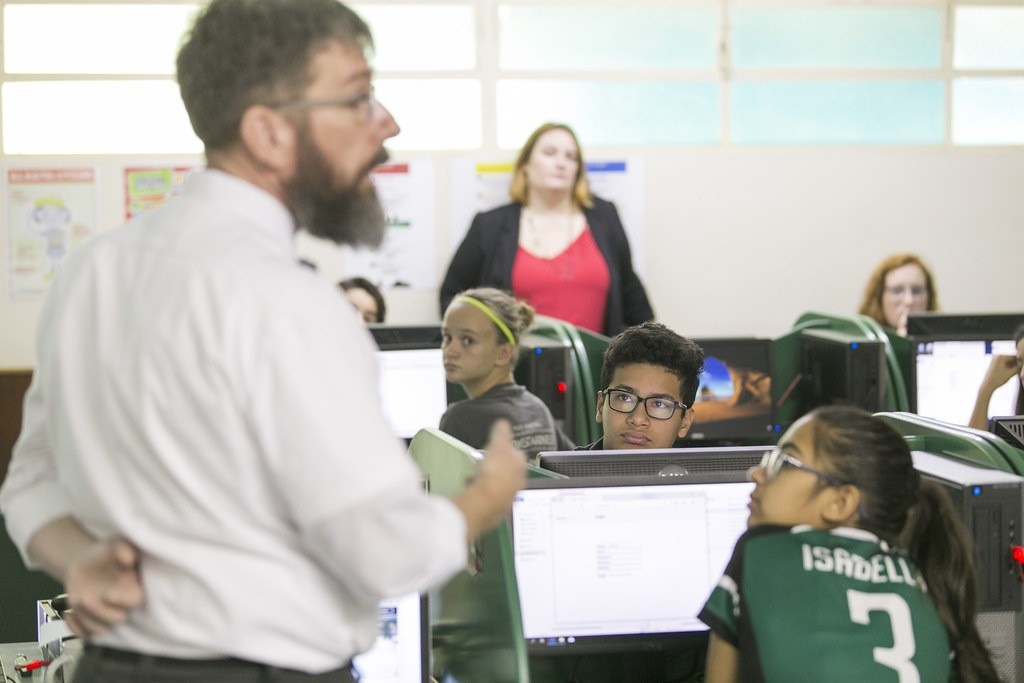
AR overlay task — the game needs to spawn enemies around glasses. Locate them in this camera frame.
[602,389,687,421]
[268,83,375,124]
[760,448,843,486]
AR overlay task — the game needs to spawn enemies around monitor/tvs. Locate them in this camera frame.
[350,328,787,683]
[905,311,1024,451]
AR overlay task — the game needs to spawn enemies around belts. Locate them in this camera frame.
[84,647,258,667]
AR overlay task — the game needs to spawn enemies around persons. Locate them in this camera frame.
[967,324,1024,432]
[440,288,560,469]
[853,251,940,341]
[696,403,1003,683]
[1,1,527,683]
[339,278,387,324]
[438,121,656,400]
[572,322,705,453]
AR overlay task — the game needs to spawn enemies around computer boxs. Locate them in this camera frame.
[799,328,884,417]
[909,447,1024,683]
[516,333,570,430]
